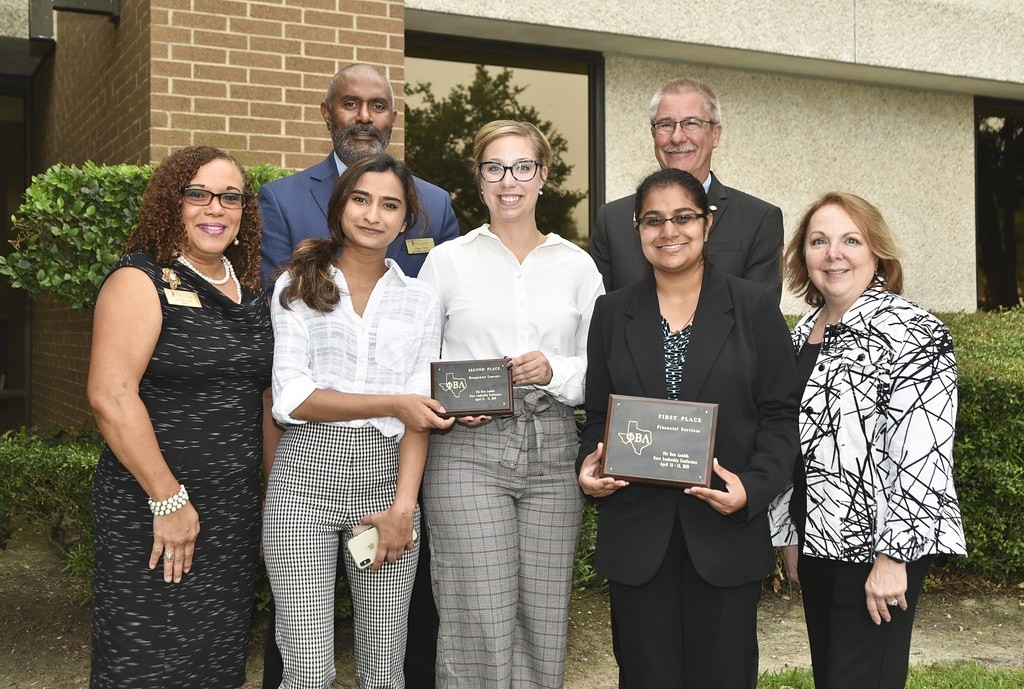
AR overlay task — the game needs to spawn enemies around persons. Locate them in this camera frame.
[416,119,607,689]
[575,167,801,689]
[87,145,280,689]
[261,60,463,689]
[766,192,970,689]
[260,153,444,689]
[591,77,785,307]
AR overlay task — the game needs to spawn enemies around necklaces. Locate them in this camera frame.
[670,308,695,332]
[180,252,243,306]
[538,230,544,244]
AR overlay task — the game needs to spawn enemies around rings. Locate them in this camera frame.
[887,599,898,606]
[164,552,175,561]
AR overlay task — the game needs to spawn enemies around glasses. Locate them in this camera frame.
[478,160,542,183]
[653,117,718,135]
[637,213,709,228]
[183,188,248,209]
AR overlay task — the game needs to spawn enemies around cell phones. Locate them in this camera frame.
[347,525,418,569]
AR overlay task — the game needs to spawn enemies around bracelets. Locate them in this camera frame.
[148,484,190,515]
